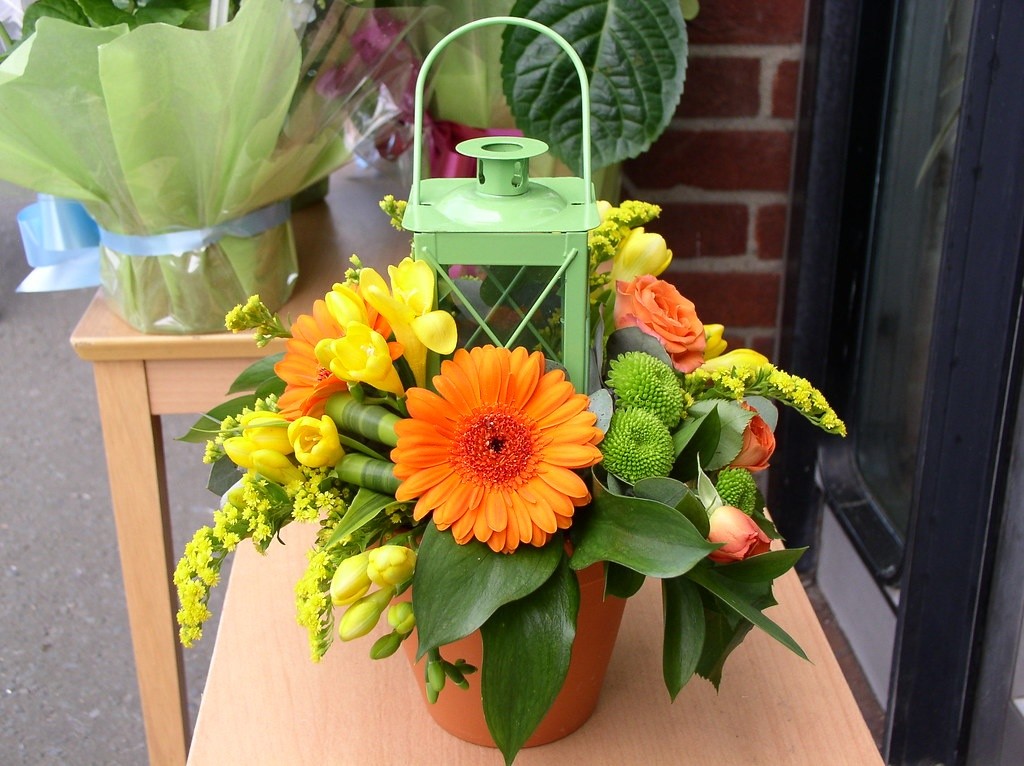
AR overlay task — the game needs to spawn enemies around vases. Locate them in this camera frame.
[365,528,627,749]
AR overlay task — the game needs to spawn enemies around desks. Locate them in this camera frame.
[184,506,882,766]
[74,148,425,766]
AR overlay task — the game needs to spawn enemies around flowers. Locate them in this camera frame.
[172,197,848,766]
